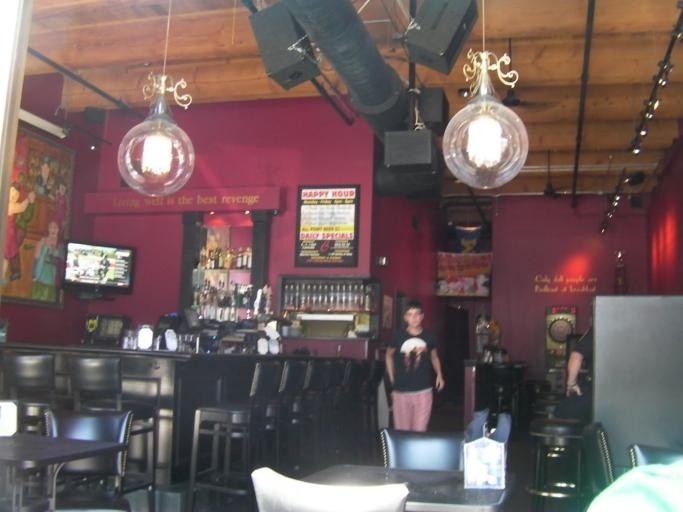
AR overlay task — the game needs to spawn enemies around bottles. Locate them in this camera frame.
[121,329,135,350]
[311,283,317,311]
[188,279,238,322]
[329,284,335,311]
[353,283,359,311]
[282,281,288,310]
[294,284,300,311]
[289,283,293,310]
[365,284,372,311]
[335,283,341,311]
[318,283,323,311]
[307,284,311,311]
[341,283,347,311]
[359,283,365,311]
[323,284,328,311]
[300,282,307,311]
[199,246,251,270]
[348,282,353,311]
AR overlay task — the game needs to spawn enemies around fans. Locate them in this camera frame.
[541,150,570,199]
[501,34,560,115]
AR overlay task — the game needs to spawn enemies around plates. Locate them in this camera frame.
[165,329,177,352]
[268,338,278,354]
[136,328,153,350]
[256,338,268,355]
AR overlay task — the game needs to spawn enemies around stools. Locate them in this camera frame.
[522,419,588,512]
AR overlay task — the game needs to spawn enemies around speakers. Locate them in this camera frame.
[406,1,479,75]
[420,87,449,136]
[249,2,320,90]
[382,129,438,175]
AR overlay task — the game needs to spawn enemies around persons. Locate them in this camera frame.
[73,251,80,278]
[2,160,67,304]
[98,255,111,280]
[383,301,445,432]
[563,320,591,398]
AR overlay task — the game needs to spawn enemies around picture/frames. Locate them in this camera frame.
[0,127,78,309]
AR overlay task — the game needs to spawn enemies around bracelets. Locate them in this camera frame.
[568,384,577,388]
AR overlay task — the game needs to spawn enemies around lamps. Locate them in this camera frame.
[629,10,682,156]
[598,168,627,235]
[117,0,196,197]
[443,0,529,188]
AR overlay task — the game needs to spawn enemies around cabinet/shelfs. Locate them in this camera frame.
[189,265,253,330]
[275,272,378,341]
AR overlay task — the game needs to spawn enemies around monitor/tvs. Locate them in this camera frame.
[61,240,137,299]
